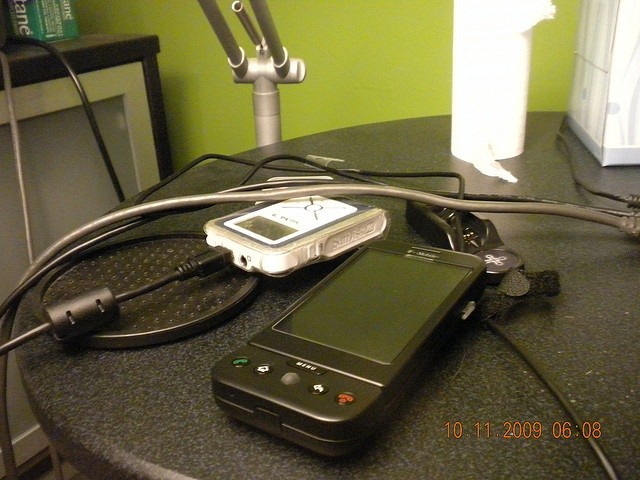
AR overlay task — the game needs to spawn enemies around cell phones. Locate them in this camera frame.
[210,240,485,458]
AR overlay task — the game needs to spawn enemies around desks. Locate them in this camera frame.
[13,110,640,479]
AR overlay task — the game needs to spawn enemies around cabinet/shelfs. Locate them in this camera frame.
[0,31,174,480]
